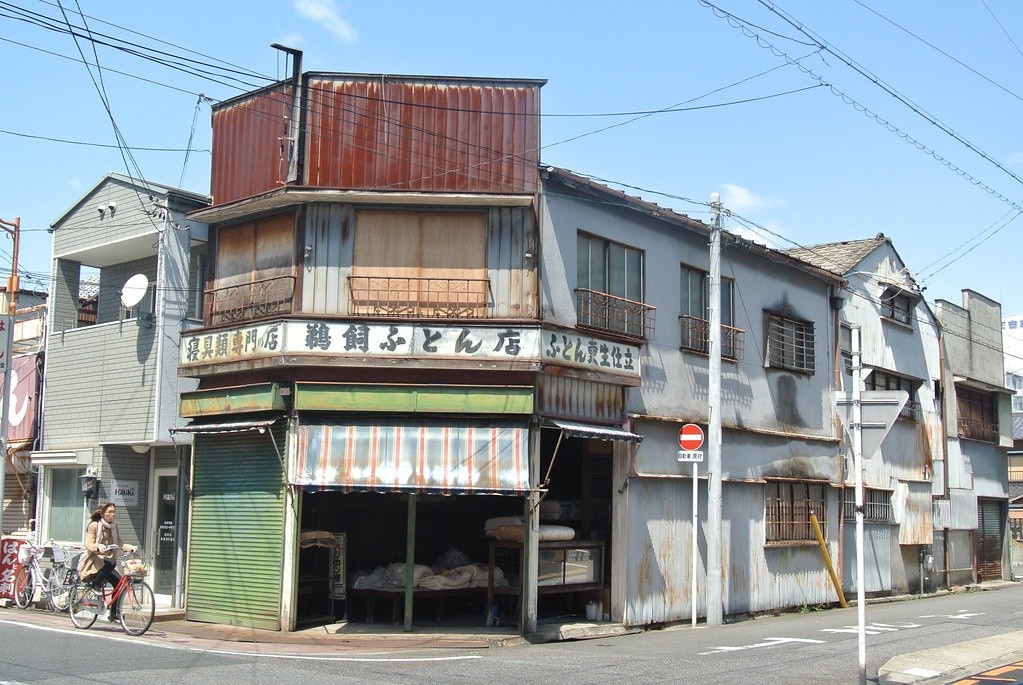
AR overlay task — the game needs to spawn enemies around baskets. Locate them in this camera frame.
[18,544,45,565]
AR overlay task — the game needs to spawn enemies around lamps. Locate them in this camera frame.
[77,468,100,508]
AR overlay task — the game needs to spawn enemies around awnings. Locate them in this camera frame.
[536,419,644,491]
[168,414,288,495]
[288,420,533,497]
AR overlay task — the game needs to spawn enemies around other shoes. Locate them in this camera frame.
[108,613,124,623]
[91,584,103,597]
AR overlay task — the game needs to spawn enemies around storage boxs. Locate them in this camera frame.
[584,584,610,618]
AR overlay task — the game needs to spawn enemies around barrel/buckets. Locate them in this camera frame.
[586,600,598,620]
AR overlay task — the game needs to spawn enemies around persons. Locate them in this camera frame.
[79,503,139,619]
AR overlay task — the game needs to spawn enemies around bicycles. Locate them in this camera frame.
[14,537,78,610]
[66,544,156,637]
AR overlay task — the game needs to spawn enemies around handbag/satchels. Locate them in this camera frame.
[122,552,147,576]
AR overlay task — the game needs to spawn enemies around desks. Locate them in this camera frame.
[344,586,488,628]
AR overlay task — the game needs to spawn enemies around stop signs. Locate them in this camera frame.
[677,424,705,451]
